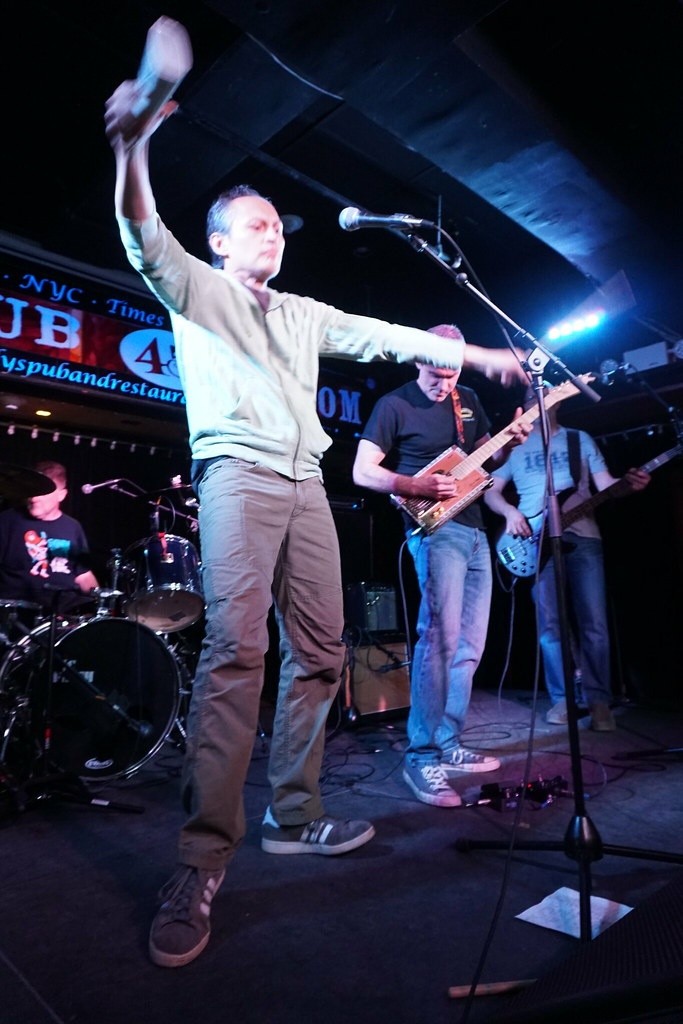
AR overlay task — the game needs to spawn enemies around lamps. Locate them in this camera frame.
[599,340,683,379]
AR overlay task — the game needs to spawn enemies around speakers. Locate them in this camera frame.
[464,876,683,1024]
[343,582,397,631]
[334,632,415,730]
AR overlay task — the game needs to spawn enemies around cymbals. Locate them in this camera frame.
[132,480,193,500]
[1,465,57,504]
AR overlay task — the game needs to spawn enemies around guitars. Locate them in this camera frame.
[391,370,596,535]
[495,443,683,578]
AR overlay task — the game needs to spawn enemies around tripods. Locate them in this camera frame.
[324,618,409,742]
[387,222,683,945]
[0,614,145,816]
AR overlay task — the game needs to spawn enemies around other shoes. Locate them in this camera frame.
[546,701,568,725]
[590,707,616,731]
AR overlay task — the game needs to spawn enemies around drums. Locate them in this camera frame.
[1,614,184,783]
[118,532,206,632]
[0,598,44,667]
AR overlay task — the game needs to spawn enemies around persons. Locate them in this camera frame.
[0,461,101,593]
[103,79,541,966]
[351,324,534,808]
[485,381,651,731]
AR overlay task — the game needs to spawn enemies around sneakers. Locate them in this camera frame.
[149,867,227,968]
[439,748,501,773]
[261,804,376,854]
[403,765,462,807]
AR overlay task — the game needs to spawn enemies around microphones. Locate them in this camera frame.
[82,479,121,494]
[150,497,161,532]
[602,363,633,385]
[339,207,435,231]
[380,661,409,674]
[128,718,155,738]
